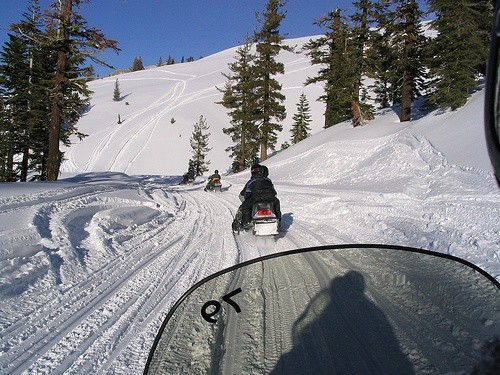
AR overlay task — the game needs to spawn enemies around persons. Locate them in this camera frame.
[207,169,221,191]
[240,166,282,228]
[240,164,262,199]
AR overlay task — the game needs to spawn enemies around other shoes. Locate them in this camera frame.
[243,220,251,228]
[277,216,282,227]
[204,188,208,192]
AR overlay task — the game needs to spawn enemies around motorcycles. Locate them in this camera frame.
[208,175,222,193]
[240,192,279,243]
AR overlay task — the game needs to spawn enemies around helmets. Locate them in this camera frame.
[341,270,367,291]
[215,170,218,174]
[331,276,346,293]
[258,166,270,176]
[250,163,261,175]
